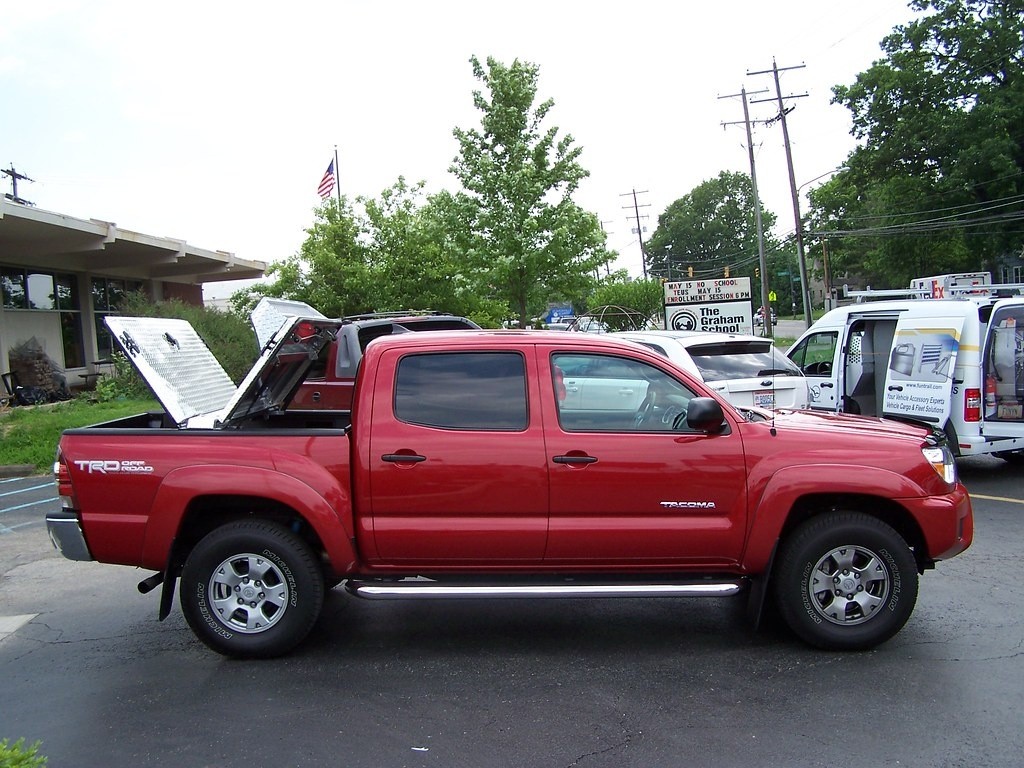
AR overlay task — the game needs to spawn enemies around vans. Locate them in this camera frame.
[906,271,993,301]
[784,283,1024,466]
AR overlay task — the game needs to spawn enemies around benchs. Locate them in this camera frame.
[78,373,107,385]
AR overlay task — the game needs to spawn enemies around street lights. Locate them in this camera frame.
[792,166,850,330]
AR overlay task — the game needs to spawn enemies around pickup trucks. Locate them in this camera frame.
[44,323,974,663]
[752,310,778,327]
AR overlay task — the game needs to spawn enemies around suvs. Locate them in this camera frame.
[550,329,810,414]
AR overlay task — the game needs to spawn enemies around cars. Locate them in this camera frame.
[335,313,608,379]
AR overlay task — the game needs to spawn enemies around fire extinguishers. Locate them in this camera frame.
[986,371,1000,406]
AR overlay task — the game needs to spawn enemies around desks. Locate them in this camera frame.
[92,358,132,383]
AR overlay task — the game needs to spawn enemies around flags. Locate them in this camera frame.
[317,158,335,200]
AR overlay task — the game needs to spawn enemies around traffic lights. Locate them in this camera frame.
[755,268,760,278]
[723,266,730,278]
